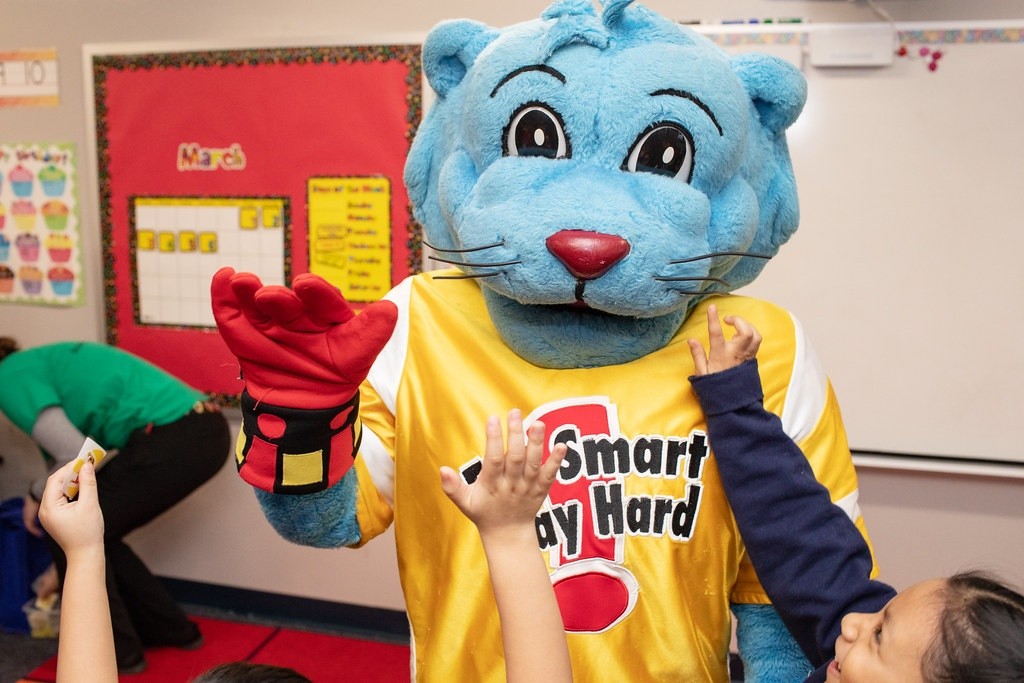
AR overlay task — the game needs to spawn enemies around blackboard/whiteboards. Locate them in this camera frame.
[680,18,1024,478]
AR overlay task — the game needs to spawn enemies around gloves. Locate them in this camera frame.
[209,266,398,496]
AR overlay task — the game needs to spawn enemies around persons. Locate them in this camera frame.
[688,303,1023,683]
[0,335,232,673]
[38,457,315,683]
[438,407,572,683]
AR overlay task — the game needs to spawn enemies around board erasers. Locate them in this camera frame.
[806,23,895,69]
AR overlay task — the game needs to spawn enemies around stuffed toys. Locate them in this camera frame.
[212,0,876,682]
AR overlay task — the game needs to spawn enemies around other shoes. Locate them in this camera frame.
[117,619,204,676]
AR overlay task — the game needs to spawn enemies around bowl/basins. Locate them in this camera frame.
[23,598,61,639]
